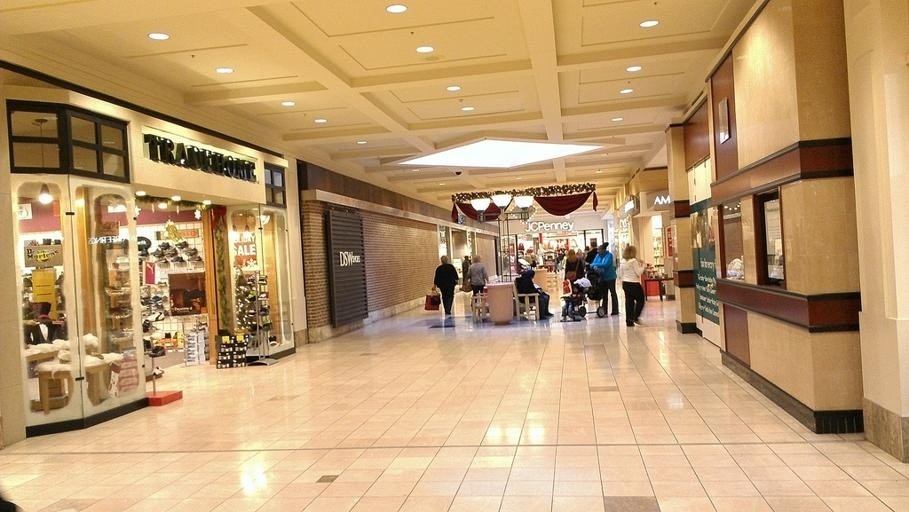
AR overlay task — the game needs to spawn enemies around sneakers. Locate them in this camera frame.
[611,312,618,314]
[142,295,165,375]
[540,312,553,320]
[627,316,639,326]
[269,336,277,346]
[247,275,273,332]
[151,241,202,263]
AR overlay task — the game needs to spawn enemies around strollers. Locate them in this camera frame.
[562,273,606,318]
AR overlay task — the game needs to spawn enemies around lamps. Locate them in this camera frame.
[35,119,169,212]
[470,194,534,282]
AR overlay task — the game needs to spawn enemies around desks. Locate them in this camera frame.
[641,274,663,302]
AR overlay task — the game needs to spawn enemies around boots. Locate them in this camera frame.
[138,247,149,256]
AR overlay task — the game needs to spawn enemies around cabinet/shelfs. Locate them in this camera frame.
[11,173,148,437]
[211,204,295,363]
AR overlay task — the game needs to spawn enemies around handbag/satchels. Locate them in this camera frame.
[566,271,576,281]
[425,293,441,310]
[462,279,473,292]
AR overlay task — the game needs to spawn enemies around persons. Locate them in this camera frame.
[461,256,471,285]
[432,256,459,316]
[503,239,618,319]
[467,256,491,306]
[621,245,644,327]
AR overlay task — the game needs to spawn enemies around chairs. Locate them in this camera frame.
[470,280,540,324]
